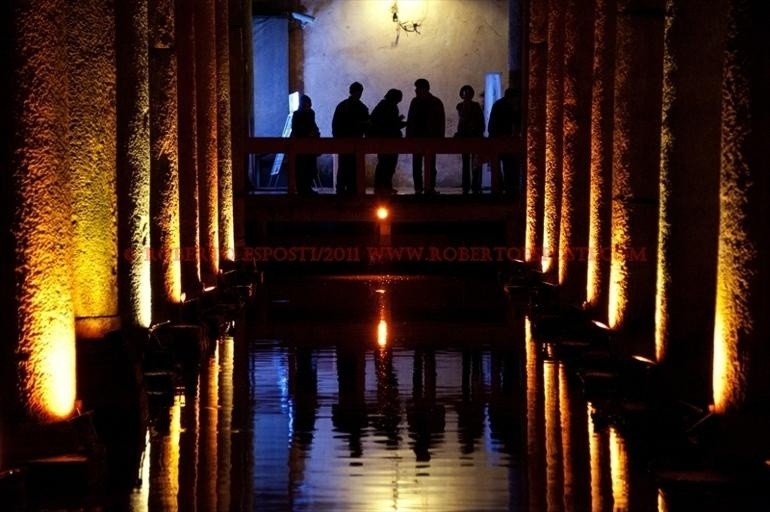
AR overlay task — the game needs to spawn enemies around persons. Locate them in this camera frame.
[331,339,372,458]
[371,346,403,449]
[487,87,517,196]
[405,78,446,195]
[286,93,326,195]
[452,83,485,195]
[277,340,320,479]
[407,348,448,462]
[368,87,407,195]
[455,354,487,452]
[330,79,370,195]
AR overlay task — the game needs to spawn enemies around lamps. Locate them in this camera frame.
[392,0,429,36]
